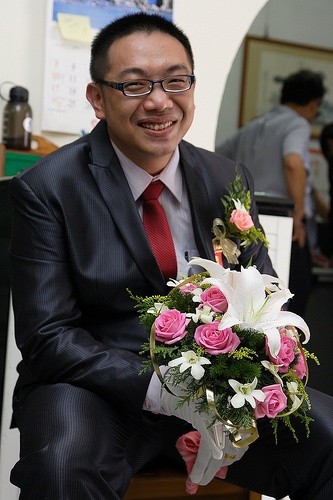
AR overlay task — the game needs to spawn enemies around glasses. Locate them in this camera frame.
[93,75,197,98]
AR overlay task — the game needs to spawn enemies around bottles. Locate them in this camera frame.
[0,80,32,151]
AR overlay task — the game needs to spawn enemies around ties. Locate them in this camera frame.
[140,179,178,294]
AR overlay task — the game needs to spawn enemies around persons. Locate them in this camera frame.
[6,13,333,500]
[313,123,333,264]
[214,69,323,325]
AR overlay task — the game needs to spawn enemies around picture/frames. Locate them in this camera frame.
[239,35,333,155]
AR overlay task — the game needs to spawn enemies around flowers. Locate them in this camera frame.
[210,173,270,265]
[126,257,321,494]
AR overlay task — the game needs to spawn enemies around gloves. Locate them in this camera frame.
[143,364,229,486]
